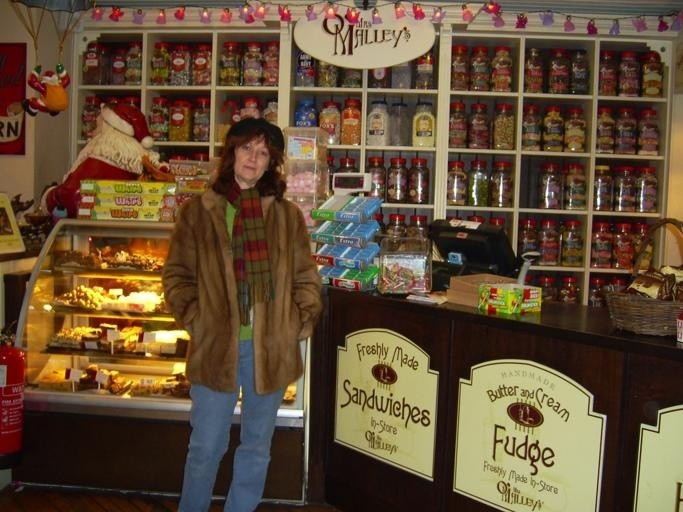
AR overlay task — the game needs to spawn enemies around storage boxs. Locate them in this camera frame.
[444,268,545,315]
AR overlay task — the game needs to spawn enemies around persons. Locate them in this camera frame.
[161,117,322,511]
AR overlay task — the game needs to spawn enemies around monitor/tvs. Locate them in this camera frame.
[429,218,519,278]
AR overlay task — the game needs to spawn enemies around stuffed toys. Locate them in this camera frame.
[42,103,175,219]
[7,0,97,117]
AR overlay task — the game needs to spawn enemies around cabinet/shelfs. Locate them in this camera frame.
[69,1,285,314]
[307,190,387,293]
[446,9,673,310]
[7,220,315,508]
[282,6,442,270]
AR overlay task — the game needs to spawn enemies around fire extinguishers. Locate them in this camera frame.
[0,319,25,455]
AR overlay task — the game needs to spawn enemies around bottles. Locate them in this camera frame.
[294,101,661,156]
[446,160,514,209]
[51,203,67,226]
[366,101,436,147]
[372,216,652,268]
[294,49,362,88]
[369,51,434,90]
[168,153,208,164]
[593,164,659,214]
[449,101,516,149]
[80,43,142,87]
[535,162,588,209]
[325,160,430,204]
[78,95,276,143]
[523,107,587,151]
[521,274,625,308]
[597,107,659,155]
[451,42,664,98]
[294,101,362,145]
[219,42,280,88]
[151,42,211,86]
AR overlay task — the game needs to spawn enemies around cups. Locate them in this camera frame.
[676,318,683,343]
